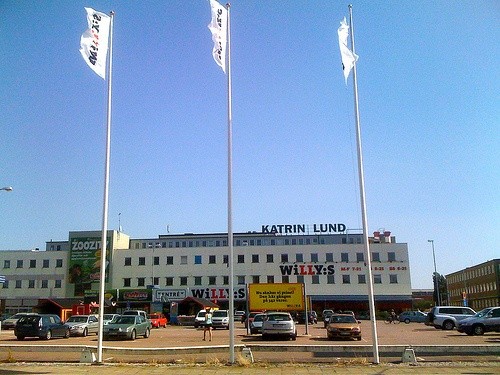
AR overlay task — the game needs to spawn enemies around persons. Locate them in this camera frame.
[390,309,395,324]
[202,309,213,341]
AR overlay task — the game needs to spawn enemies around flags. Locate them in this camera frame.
[208,0,227,73]
[337,16,359,85]
[78,6,111,79]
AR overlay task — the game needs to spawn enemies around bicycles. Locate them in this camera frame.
[384,314,400,324]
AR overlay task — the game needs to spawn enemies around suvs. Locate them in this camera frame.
[194,309,230,330]
[322,310,334,320]
[427,306,478,330]
[458,307,500,336]
[308,311,318,324]
[14,314,70,341]
[262,311,297,341]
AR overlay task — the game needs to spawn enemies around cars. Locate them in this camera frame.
[3,313,38,330]
[250,314,264,335]
[103,314,120,326]
[324,314,362,341]
[146,313,168,328]
[63,315,100,337]
[401,311,428,324]
[103,315,150,340]
[245,312,261,328]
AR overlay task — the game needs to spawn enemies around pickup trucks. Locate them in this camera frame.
[125,310,146,319]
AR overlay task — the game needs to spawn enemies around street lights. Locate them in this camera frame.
[148,244,161,285]
[428,240,440,306]
